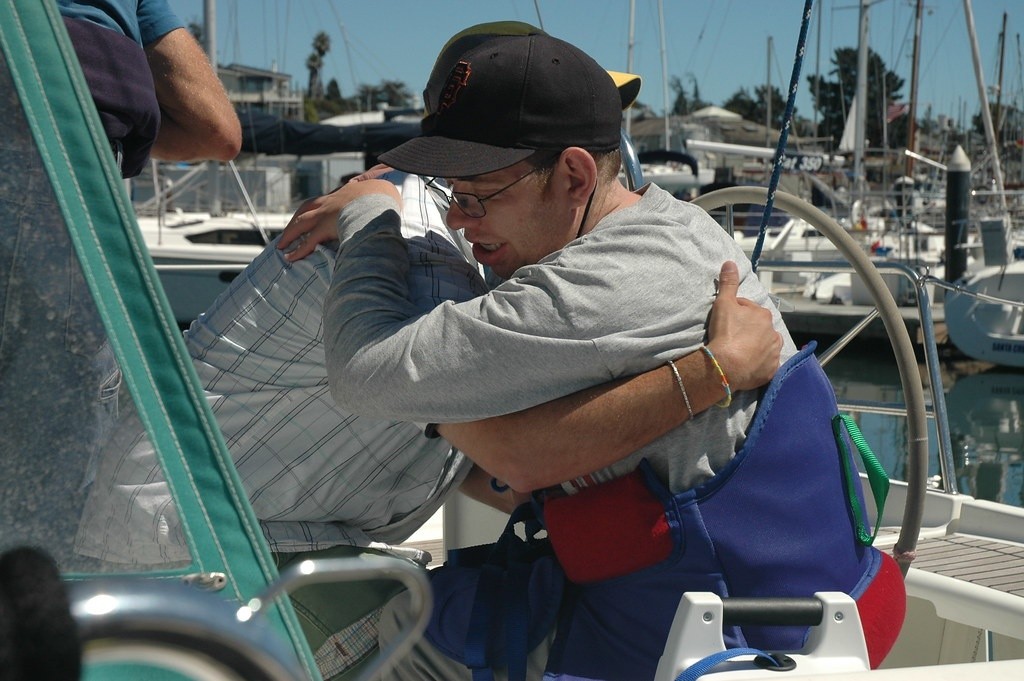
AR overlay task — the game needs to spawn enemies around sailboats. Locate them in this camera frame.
[125,2,1024,388]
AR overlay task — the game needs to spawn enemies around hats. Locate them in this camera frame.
[377,31,622,178]
[424,20,641,115]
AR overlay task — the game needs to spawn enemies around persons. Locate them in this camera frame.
[277,33,906,681]
[71,20,785,680]
[0,0,240,569]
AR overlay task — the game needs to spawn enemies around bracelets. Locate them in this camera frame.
[669,360,694,420]
[699,346,732,408]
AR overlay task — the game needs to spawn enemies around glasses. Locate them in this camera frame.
[424,166,544,220]
